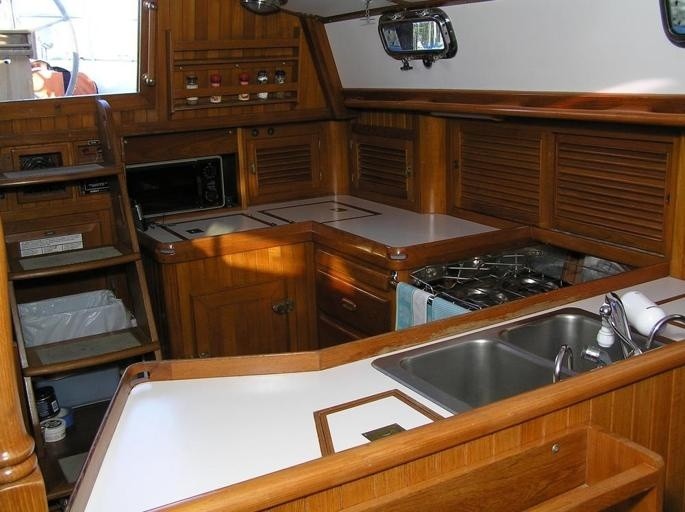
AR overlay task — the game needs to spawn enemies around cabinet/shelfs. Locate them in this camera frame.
[64,260,685,501]
[309,215,526,349]
[142,210,316,356]
[1,99,161,497]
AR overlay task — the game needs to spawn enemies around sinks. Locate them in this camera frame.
[474,307,677,373]
[371,333,573,416]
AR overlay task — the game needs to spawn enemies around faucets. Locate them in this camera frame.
[605,291,633,358]
[552,343,573,384]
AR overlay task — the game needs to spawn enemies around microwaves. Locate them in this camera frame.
[122,153,228,218]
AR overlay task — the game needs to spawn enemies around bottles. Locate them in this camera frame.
[274,71,286,99]
[185,75,199,104]
[210,74,222,103]
[620,290,667,337]
[256,71,269,99]
[238,72,250,101]
